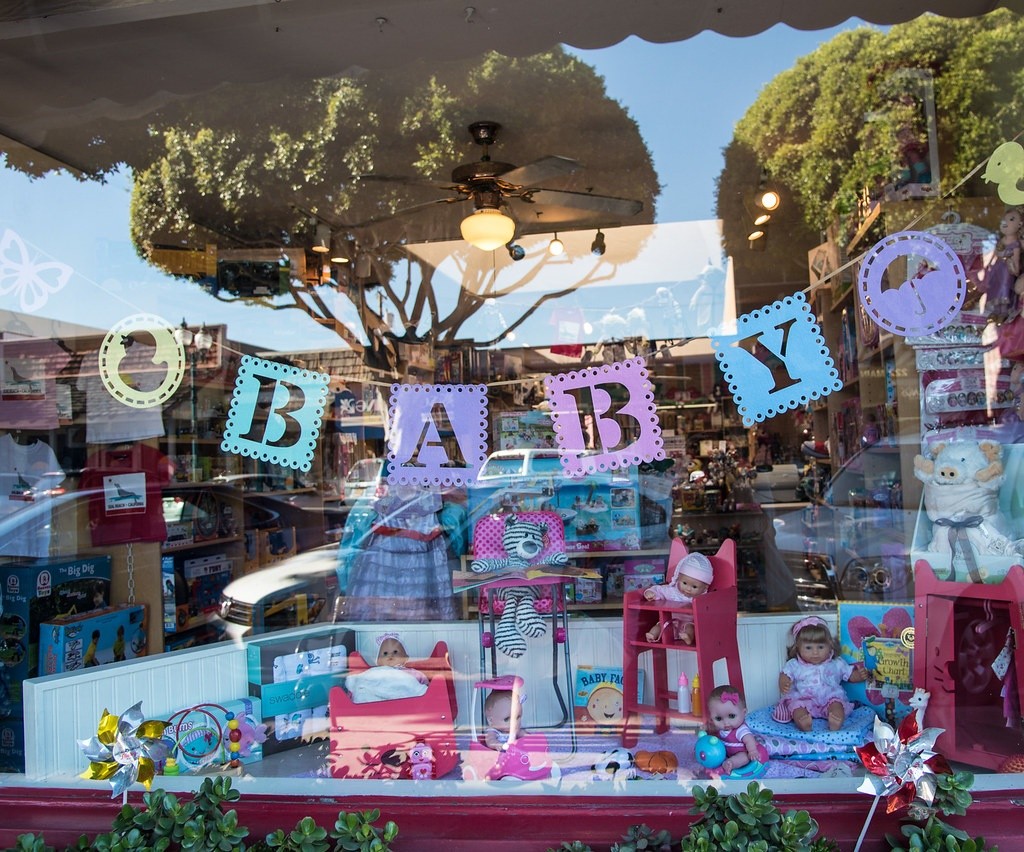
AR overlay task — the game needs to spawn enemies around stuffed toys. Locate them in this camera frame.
[471,514,568,659]
[915,438,1022,555]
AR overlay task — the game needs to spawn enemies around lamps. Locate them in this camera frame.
[754,173,780,210]
[354,250,371,278]
[549,231,563,255]
[311,224,331,253]
[743,191,771,226]
[505,236,525,261]
[330,230,351,262]
[591,227,606,255]
[741,215,765,241]
[461,189,515,251]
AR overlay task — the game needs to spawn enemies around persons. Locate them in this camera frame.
[700,685,761,774]
[645,552,713,644]
[485,690,527,751]
[345,633,430,703]
[772,616,868,730]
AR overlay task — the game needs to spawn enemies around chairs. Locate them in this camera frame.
[622,537,746,747]
[475,510,579,764]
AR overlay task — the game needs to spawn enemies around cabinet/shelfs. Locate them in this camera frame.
[1,199,1023,780]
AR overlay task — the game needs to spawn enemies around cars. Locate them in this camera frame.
[0,420,1024,733]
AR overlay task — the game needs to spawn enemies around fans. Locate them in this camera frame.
[343,121,643,231]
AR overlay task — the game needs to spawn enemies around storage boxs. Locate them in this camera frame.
[268,440,296,490]
[39,601,148,675]
[575,568,601,604]
[700,440,726,456]
[1,719,25,772]
[247,628,355,754]
[163,634,195,651]
[259,528,296,568]
[182,553,234,580]
[623,559,665,592]
[159,558,176,633]
[606,565,623,597]
[0,552,113,719]
[462,455,641,552]
[197,456,240,484]
[242,423,266,492]
[809,242,853,291]
[244,528,259,574]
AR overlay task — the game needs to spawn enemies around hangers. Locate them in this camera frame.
[118,335,136,350]
[102,442,135,453]
[8,430,36,446]
[51,320,76,358]
